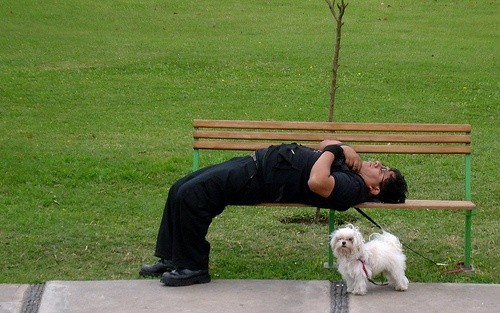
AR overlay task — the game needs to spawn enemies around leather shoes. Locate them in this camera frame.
[139,258,176,276]
[160,264,210,286]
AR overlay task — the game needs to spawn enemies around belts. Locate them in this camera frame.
[251,155,257,167]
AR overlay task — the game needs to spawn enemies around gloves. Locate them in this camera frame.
[324,144,344,159]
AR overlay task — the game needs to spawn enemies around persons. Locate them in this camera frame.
[138,139,408,287]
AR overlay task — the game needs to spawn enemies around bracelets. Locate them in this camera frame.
[338,142,347,147]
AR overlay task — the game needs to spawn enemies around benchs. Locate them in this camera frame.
[193,118,476,266]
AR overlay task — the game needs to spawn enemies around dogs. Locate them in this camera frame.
[329,223,410,295]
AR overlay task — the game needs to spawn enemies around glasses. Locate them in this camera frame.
[380,165,389,182]
